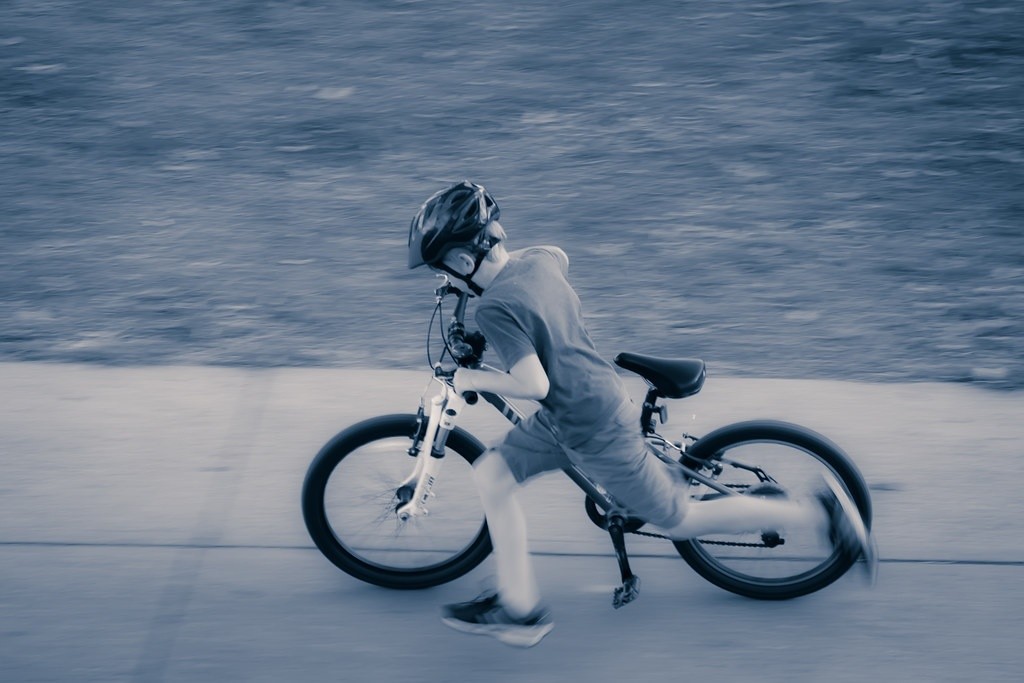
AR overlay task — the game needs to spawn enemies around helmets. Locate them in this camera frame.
[408,180,501,269]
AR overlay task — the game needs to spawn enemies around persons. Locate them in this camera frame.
[408,180,877,648]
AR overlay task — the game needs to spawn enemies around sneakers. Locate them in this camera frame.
[443,589,557,647]
[816,470,879,587]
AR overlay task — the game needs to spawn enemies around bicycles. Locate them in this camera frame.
[298,271,873,613]
